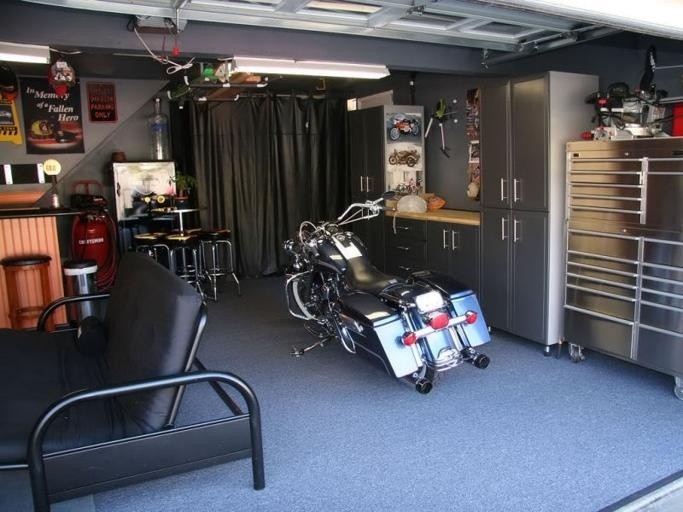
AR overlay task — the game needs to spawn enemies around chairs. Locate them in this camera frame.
[1,252,265,512]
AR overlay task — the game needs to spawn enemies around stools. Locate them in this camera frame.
[1,255,98,335]
[133,224,243,311]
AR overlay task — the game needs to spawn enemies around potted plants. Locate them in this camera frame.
[169,170,198,210]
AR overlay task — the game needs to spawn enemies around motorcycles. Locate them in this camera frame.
[280,189,490,394]
[390,118,419,141]
[395,178,424,194]
[389,149,419,167]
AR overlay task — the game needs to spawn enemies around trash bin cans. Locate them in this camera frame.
[60,258,103,322]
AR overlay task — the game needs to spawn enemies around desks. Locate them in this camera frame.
[151,206,210,282]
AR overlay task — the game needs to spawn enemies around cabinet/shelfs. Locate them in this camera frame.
[347,105,426,274]
[479,70,600,354]
[427,220,479,302]
[384,216,426,280]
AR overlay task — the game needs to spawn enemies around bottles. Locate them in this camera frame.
[50,175,62,210]
[149,97,170,161]
